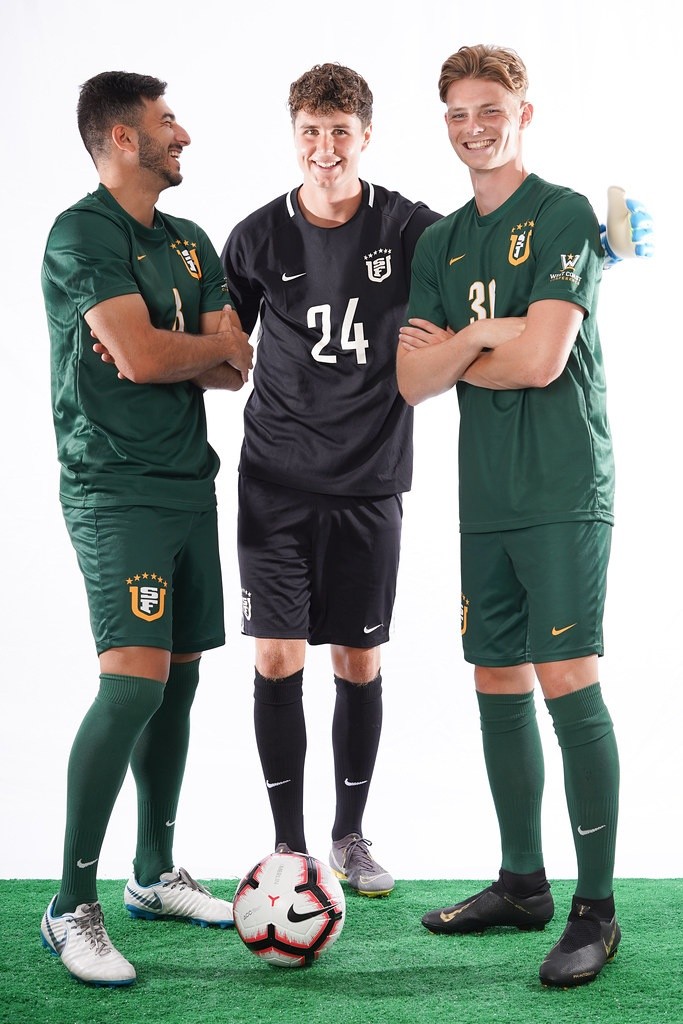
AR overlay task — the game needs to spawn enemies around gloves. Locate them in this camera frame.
[599,187,652,271]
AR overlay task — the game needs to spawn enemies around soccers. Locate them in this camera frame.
[231,851,346,968]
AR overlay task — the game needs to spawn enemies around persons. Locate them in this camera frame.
[39,73,252,987]
[214,60,652,899]
[395,44,622,986]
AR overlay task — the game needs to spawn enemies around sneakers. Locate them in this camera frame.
[123,865,238,929]
[539,889,622,989]
[420,877,555,936]
[329,833,394,898]
[39,890,136,985]
